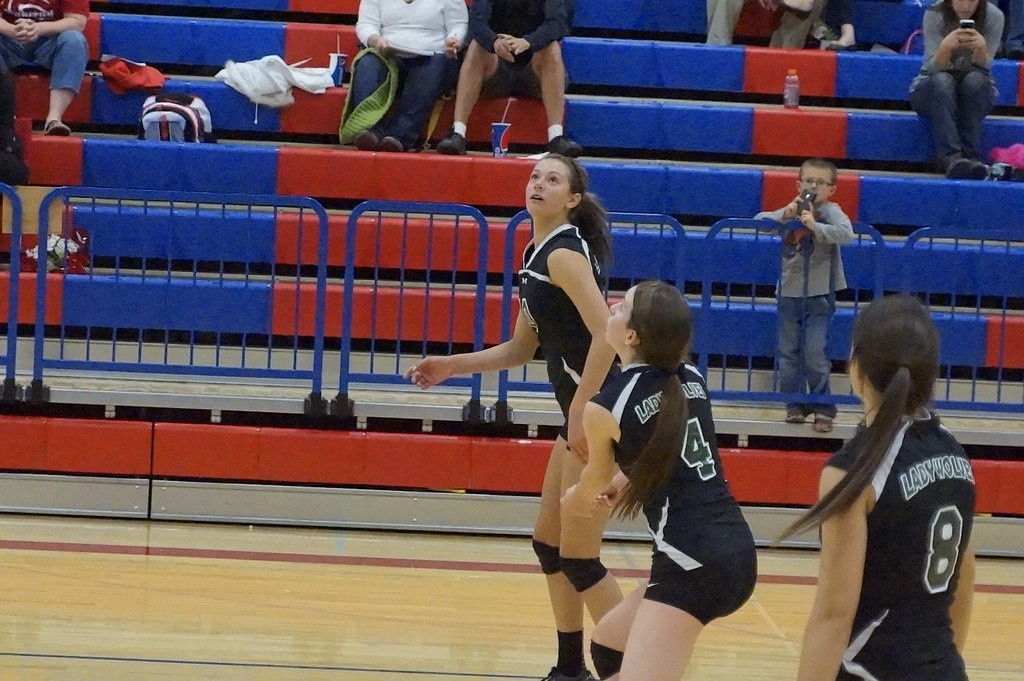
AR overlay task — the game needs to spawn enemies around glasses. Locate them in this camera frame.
[799,178,833,188]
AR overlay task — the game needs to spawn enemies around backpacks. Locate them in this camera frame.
[137,94,216,144]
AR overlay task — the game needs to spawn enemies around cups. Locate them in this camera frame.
[491,123,512,159]
[14,117,33,141]
[328,53,349,87]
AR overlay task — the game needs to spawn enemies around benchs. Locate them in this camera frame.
[0,0,1024,379]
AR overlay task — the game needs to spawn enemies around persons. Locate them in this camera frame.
[706,0,859,51]
[908,0,1024,180]
[754,157,853,433]
[0,53,30,196]
[798,294,975,681]
[403,153,638,681]
[354,0,583,159]
[560,281,757,681]
[0,0,90,137]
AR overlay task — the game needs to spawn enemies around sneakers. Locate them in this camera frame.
[538,666,595,681]
[814,412,832,432]
[438,124,467,154]
[549,131,582,156]
[787,402,805,422]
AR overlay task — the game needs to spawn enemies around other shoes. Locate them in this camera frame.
[821,38,857,51]
[950,159,988,179]
[44,117,71,136]
[382,135,410,151]
[355,131,383,150]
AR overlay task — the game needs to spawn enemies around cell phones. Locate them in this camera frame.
[960,20,975,28]
[796,189,818,214]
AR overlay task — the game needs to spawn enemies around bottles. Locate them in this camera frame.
[784,69,799,110]
